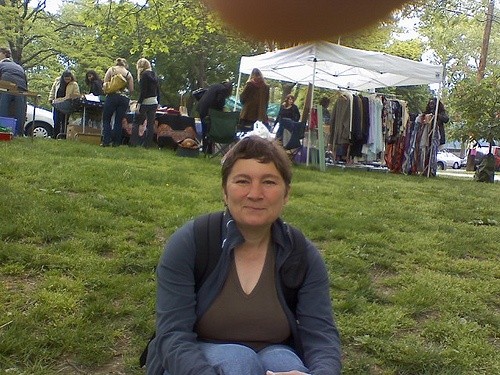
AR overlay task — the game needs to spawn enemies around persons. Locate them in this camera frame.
[145,135,343,375]
[100,57,134,147]
[196,81,233,154]
[319,95,331,125]
[127,58,161,149]
[47,70,81,139]
[278,94,301,121]
[422,98,449,145]
[78,70,103,128]
[0,48,27,136]
[239,68,270,134]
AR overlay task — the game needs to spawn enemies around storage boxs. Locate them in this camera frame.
[66,125,102,144]
[0,117,18,136]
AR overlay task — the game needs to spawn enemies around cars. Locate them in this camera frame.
[435,158,448,170]
[24,101,54,138]
[437,152,461,169]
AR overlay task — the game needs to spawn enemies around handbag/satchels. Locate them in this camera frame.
[192,88,209,101]
[103,67,127,94]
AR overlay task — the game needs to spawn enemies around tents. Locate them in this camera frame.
[228,39,444,178]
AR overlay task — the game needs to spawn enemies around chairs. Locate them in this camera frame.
[276,117,307,165]
[203,108,240,159]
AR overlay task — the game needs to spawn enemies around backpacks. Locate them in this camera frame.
[137,212,309,369]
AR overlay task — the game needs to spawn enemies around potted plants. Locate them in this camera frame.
[0,127,14,141]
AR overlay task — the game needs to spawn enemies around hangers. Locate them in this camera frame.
[328,89,348,100]
[345,91,406,102]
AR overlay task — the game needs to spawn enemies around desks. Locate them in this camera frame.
[122,113,195,151]
[0,88,40,143]
[52,97,104,144]
